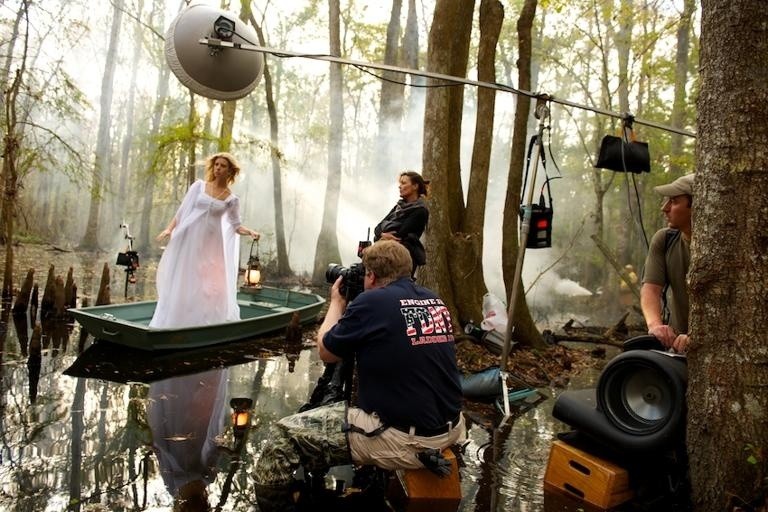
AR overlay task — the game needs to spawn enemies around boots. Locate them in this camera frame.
[252,483,296,512]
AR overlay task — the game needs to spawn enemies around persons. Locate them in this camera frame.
[146,365,230,512]
[375,171,429,282]
[149,152,260,329]
[639,173,695,355]
[253,241,466,512]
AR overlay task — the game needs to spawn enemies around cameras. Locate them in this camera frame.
[325,263,366,301]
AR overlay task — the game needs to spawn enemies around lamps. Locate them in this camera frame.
[240,237,264,296]
[228,396,253,439]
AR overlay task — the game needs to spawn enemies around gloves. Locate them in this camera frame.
[415,449,452,478]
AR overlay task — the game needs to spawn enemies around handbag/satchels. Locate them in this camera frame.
[594,136,650,173]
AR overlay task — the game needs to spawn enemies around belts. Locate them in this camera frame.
[391,417,460,437]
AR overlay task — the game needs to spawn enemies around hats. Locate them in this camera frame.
[653,173,695,197]
